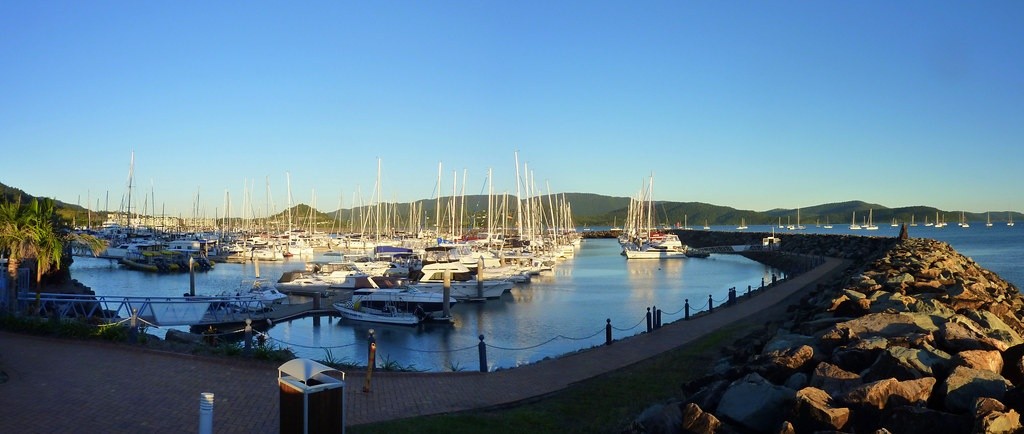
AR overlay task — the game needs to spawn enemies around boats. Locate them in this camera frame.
[332,285,458,326]
[683,248,710,258]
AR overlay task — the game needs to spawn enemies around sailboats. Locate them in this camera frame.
[683,205,1015,230]
[71,148,582,301]
[618,170,693,258]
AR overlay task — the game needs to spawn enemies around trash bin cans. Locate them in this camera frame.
[276,357,345,434]
